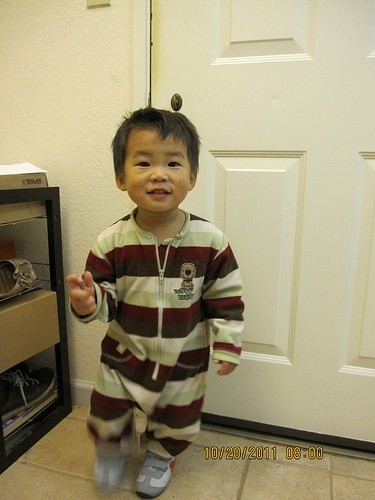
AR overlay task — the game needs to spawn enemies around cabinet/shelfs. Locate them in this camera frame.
[0,188,72,474]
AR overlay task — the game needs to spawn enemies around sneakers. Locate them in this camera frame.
[0,363,56,421]
[94,454,125,489]
[135,451,177,499]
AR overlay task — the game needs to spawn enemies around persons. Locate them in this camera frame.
[66,106,244,499]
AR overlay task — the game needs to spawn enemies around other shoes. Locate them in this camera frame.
[0,258,37,302]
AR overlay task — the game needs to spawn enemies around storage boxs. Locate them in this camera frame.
[0,290,61,374]
[0,162,47,224]
